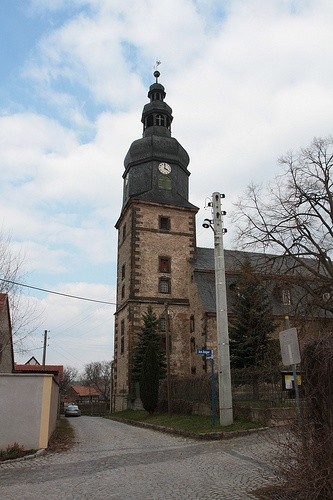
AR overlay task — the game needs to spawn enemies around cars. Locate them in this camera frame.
[65,405,81,417]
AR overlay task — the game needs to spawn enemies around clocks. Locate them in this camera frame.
[159,162,171,175]
